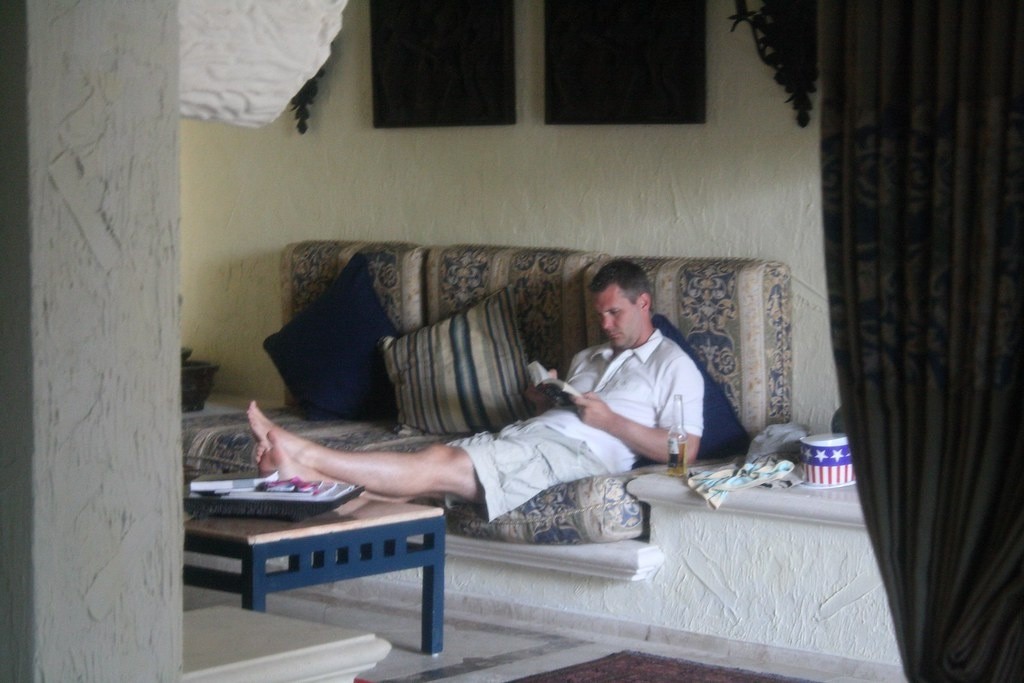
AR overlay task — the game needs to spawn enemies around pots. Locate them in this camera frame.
[181,347,220,412]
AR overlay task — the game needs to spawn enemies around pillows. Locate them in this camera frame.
[376,283,538,435]
[262,250,405,425]
[631,313,751,470]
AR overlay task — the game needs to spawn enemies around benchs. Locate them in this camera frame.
[178,241,793,546]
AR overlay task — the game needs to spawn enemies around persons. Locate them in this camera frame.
[245,258,705,525]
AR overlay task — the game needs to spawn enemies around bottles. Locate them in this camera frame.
[667,394,688,477]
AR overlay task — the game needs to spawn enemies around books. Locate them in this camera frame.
[527,358,584,407]
[190,468,355,502]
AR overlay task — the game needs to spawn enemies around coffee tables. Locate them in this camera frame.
[184,494,446,656]
